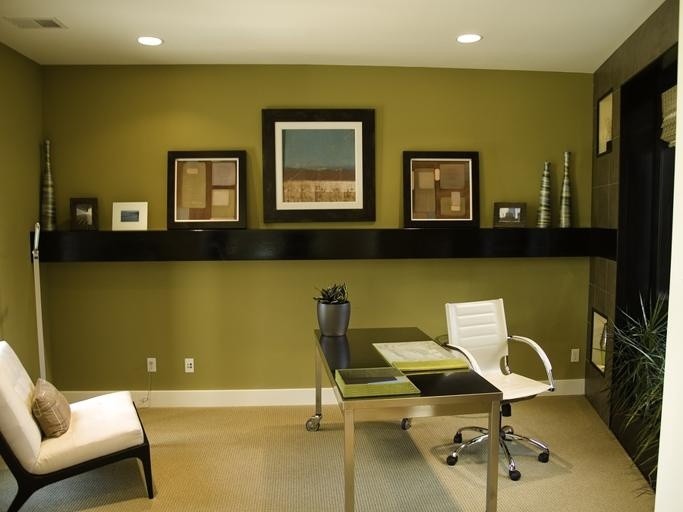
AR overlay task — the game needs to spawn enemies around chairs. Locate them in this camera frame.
[434,296,557,480]
[0,341,158,512]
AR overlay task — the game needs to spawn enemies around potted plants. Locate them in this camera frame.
[310,279,356,341]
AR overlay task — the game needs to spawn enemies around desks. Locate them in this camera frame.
[305,327,501,512]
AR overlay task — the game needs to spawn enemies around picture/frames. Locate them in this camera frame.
[165,148,247,230]
[593,86,616,158]
[587,312,610,375]
[258,105,377,223]
[69,195,98,230]
[399,148,481,229]
[491,200,527,230]
[111,200,148,232]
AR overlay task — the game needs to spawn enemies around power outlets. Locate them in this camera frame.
[182,356,196,375]
[146,354,157,375]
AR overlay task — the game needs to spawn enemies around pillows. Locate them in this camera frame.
[30,373,74,438]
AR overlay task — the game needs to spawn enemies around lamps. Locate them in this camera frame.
[25,221,51,386]
